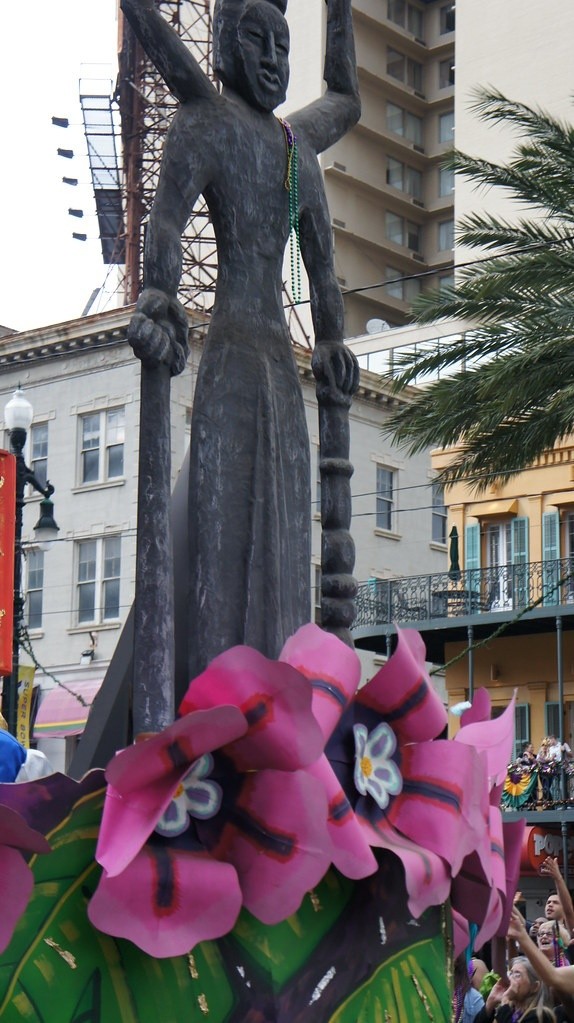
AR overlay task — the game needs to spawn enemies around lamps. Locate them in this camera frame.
[490,662,499,682]
[80,649,94,665]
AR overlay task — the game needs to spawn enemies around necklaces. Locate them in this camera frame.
[278,118,301,305]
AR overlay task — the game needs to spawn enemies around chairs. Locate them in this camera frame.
[468,584,497,614]
[393,589,426,621]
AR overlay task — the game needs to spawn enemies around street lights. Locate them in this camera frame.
[5,389,62,734]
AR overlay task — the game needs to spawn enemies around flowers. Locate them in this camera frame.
[86,619,527,959]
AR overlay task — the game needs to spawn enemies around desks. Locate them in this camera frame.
[432,590,476,616]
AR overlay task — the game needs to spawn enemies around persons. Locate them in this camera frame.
[64,0,361,779]
[460,730,573,1022]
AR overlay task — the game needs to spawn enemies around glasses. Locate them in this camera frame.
[507,971,530,981]
[537,932,553,937]
